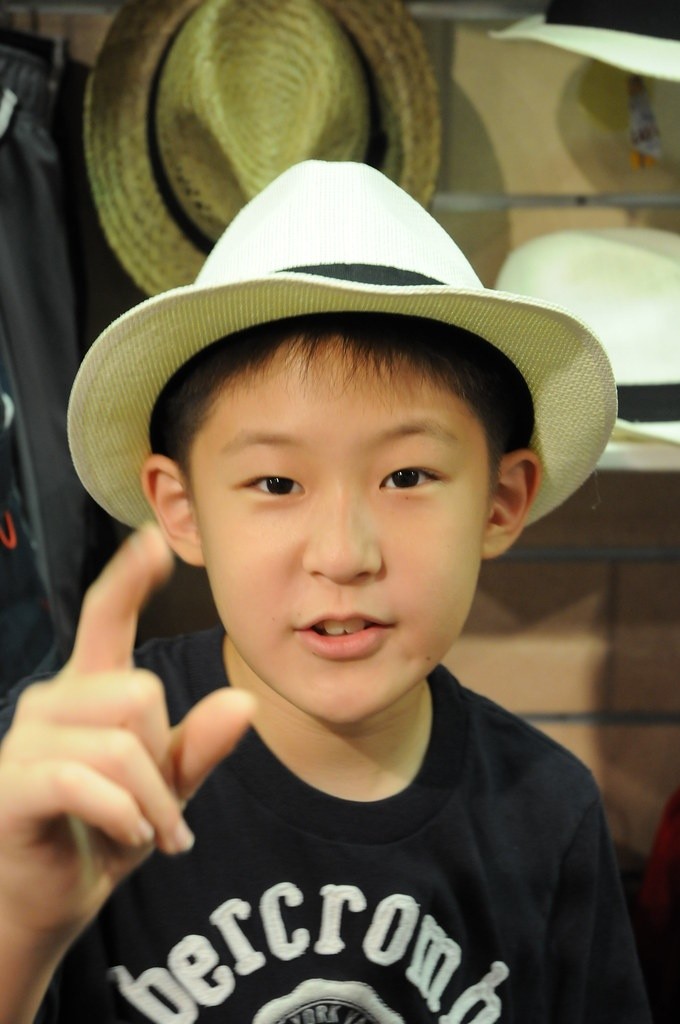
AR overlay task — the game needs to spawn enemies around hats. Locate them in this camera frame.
[66,159,620,526]
[496,227,680,446]
[84,0,442,295]
[485,0,680,82]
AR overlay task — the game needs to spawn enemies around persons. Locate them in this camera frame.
[0,158,648,1024]
